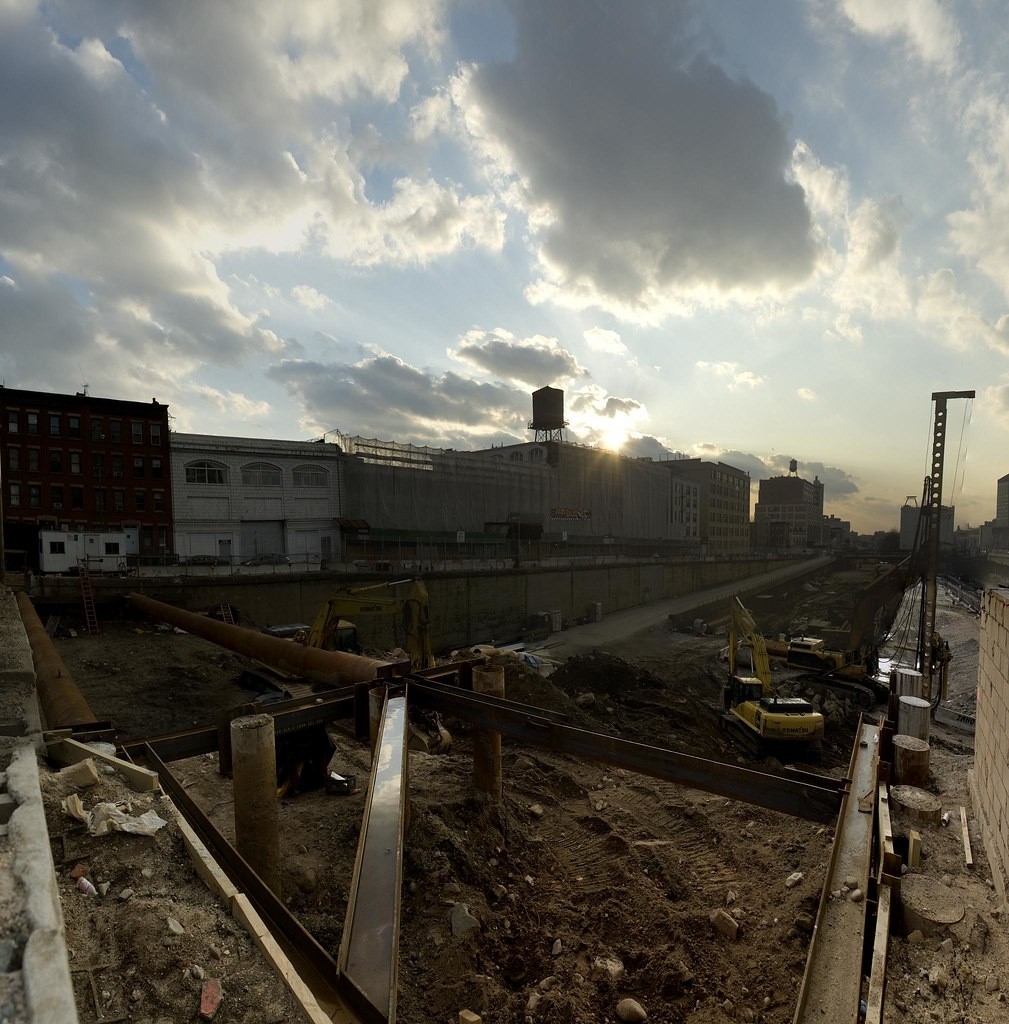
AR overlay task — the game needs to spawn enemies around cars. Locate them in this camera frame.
[178,554,221,565]
[240,553,290,567]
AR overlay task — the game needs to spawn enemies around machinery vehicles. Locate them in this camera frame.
[716,595,826,765]
[230,578,452,756]
[785,634,891,713]
